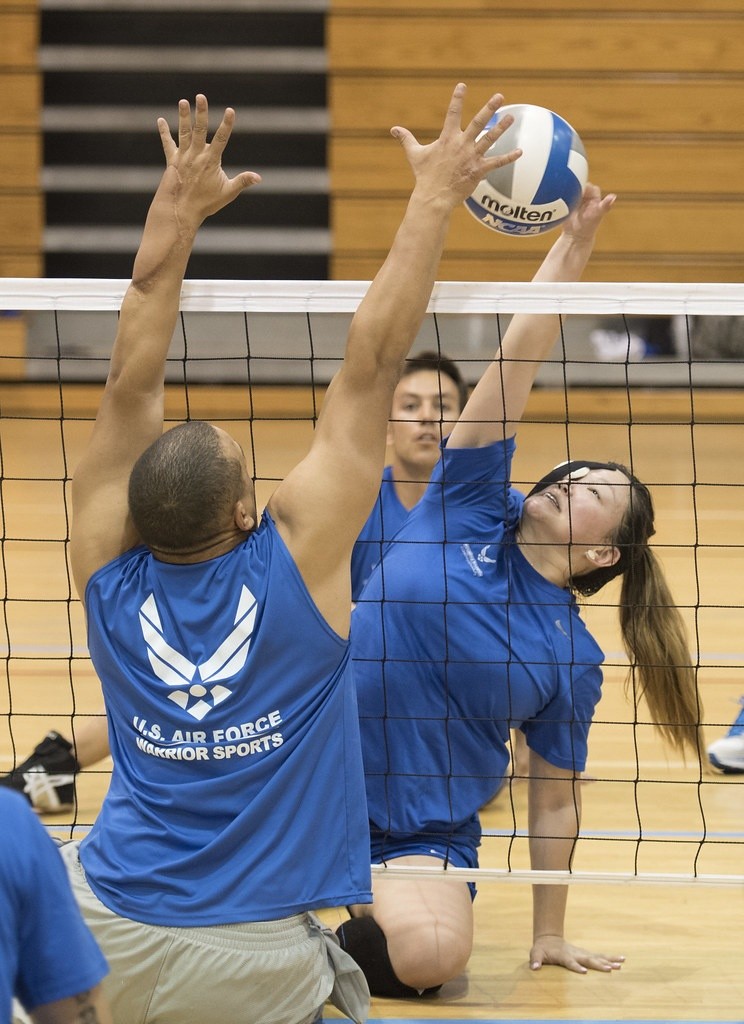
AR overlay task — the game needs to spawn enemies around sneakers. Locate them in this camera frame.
[707,708,744,774]
[0,730,80,813]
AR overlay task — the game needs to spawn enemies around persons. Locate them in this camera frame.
[0,80,744,1024]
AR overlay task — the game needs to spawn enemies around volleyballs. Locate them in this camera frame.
[460,102,592,237]
